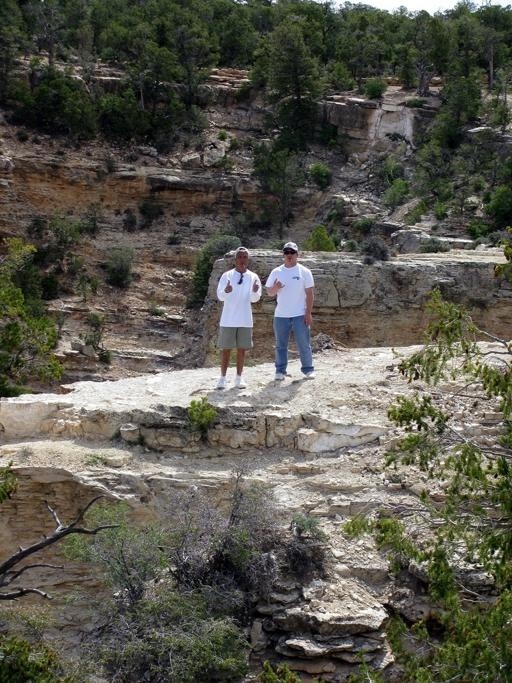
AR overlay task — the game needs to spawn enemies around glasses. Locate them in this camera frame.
[284,250,297,254]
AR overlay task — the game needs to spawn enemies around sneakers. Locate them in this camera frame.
[275,373,284,380]
[306,371,316,378]
[235,374,247,389]
[217,376,226,389]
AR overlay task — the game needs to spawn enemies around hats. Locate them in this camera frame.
[283,242,298,252]
[235,247,249,259]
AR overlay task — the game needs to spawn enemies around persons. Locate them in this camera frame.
[265,242,315,380]
[216,247,261,389]
